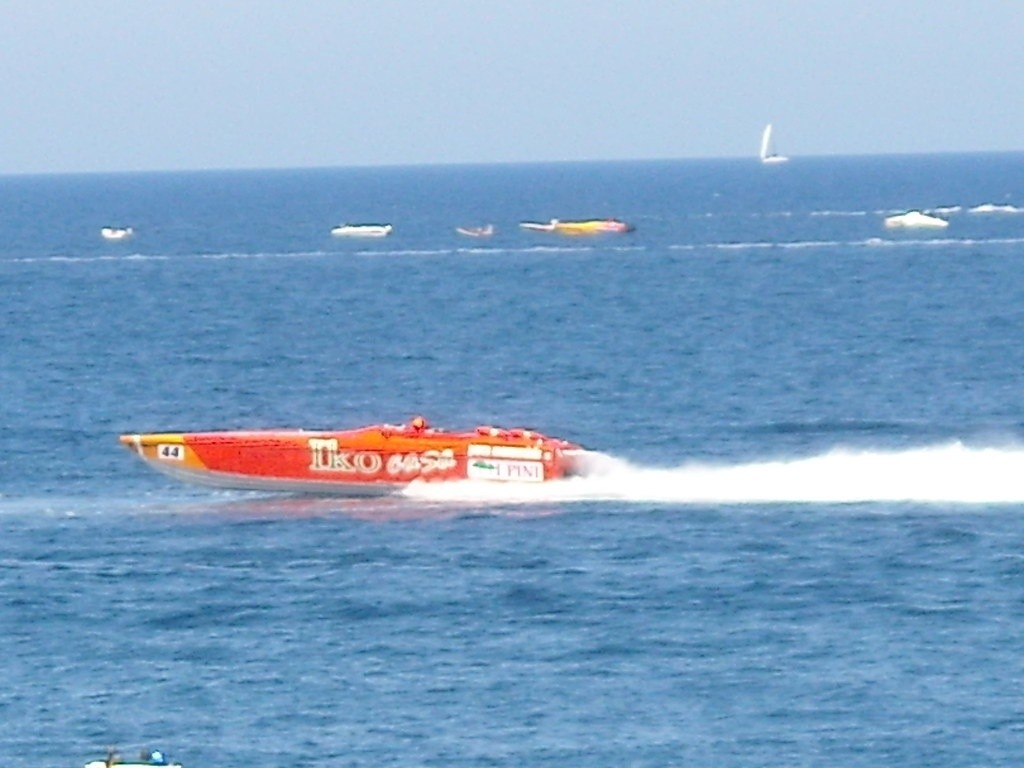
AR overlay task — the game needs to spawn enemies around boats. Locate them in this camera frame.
[118,415,597,498]
[328,223,391,239]
[100,225,132,240]
[517,215,636,235]
[455,223,493,237]
[883,209,950,227]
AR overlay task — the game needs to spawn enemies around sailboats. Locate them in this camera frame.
[760,124,790,164]
